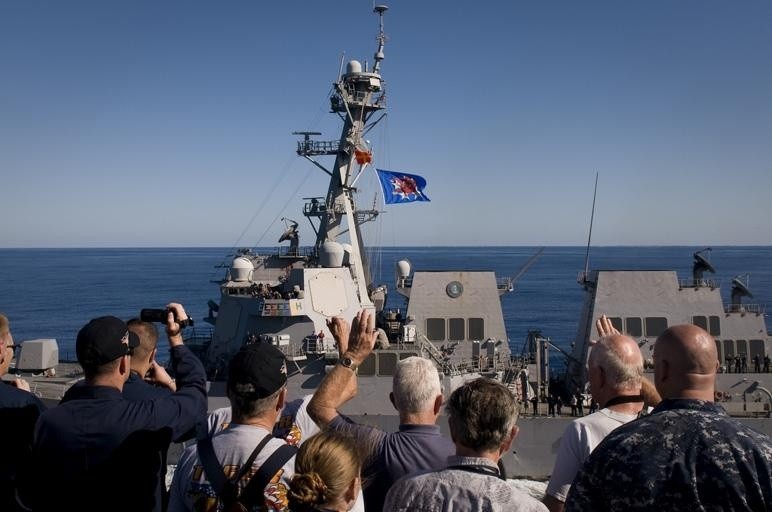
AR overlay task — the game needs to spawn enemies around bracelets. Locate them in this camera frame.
[165,379,176,385]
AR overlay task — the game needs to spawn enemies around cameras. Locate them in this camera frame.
[140,306,178,326]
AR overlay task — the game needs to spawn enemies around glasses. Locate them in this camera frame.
[7,343,22,357]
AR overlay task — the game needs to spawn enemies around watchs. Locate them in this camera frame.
[335,356,358,373]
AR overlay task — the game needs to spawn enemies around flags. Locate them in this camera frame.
[376,167,430,205]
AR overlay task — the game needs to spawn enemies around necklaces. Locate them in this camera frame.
[600,395,646,406]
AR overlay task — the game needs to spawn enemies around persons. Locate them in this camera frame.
[240,331,273,346]
[0,316,48,511]
[376,306,400,349]
[34,300,207,510]
[310,329,318,351]
[308,307,458,510]
[291,430,364,512]
[319,330,325,351]
[578,325,771,512]
[383,379,550,512]
[166,342,299,512]
[247,281,304,317]
[59,320,177,399]
[725,352,770,373]
[192,315,353,452]
[540,315,662,511]
[515,372,597,417]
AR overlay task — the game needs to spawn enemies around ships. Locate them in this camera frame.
[6,6,771,476]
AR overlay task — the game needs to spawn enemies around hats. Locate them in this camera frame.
[227,341,288,400]
[75,315,140,366]
